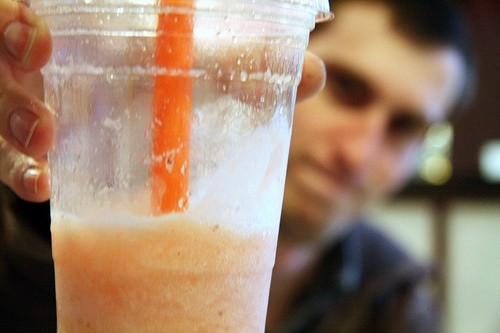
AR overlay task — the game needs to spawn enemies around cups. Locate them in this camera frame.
[30,0,332,332]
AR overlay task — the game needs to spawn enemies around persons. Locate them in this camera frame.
[0,0,480,332]
